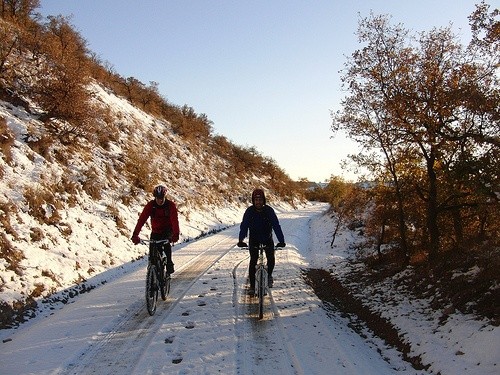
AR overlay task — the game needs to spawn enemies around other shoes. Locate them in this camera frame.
[268,282,273,287]
[167,264,174,273]
[247,290,254,294]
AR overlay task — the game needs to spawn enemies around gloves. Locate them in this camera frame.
[172,236,178,242]
[276,242,286,247]
[237,242,248,247]
[131,236,140,245]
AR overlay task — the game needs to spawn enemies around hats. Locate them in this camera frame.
[252,190,264,198]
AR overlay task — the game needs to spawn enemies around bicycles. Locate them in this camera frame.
[239,243,284,320]
[131,237,174,316]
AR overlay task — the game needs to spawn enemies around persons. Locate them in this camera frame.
[237,189,286,295]
[131,186,179,298]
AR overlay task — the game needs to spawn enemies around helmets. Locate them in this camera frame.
[153,186,167,197]
